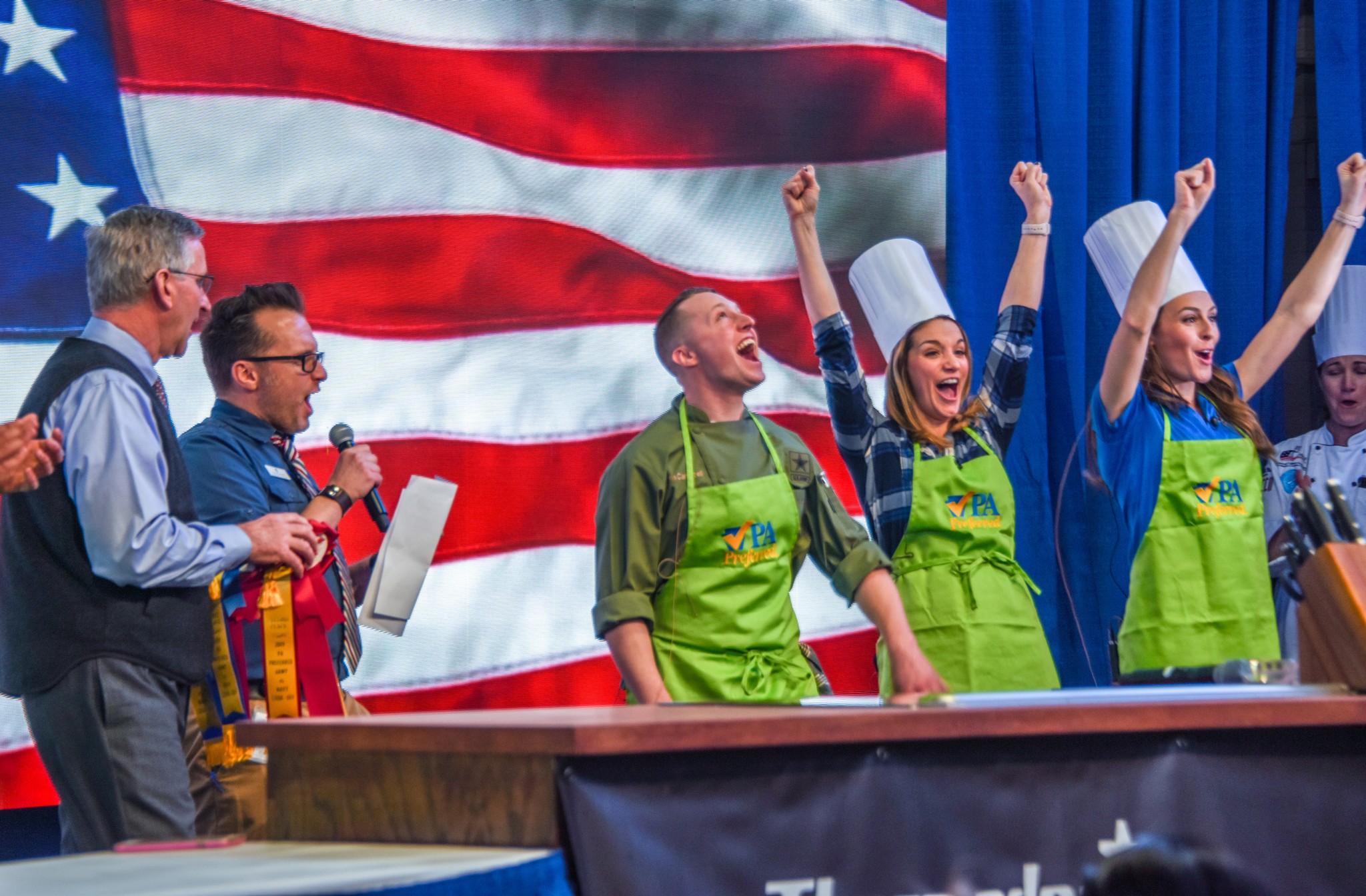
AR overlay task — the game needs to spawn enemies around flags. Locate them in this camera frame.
[0,0,946,806]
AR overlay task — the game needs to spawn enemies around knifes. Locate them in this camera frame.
[1280,475,1366,572]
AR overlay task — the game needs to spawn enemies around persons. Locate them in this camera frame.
[1263,265,1366,660]
[782,161,1060,698]
[1084,151,1366,674]
[592,287,949,705]
[0,413,64,491]
[0,205,317,856]
[176,283,382,830]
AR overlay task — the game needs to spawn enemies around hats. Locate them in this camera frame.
[1083,201,1208,318]
[849,239,955,364]
[1312,265,1366,368]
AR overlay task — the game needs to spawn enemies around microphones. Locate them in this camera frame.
[330,423,390,533]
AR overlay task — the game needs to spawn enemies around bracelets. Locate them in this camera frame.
[1332,207,1365,229]
[1022,223,1051,236]
[370,552,378,568]
[318,484,353,517]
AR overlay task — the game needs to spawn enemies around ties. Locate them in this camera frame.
[154,377,167,410]
[270,434,364,675]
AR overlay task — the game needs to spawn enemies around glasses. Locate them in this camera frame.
[146,267,214,295]
[237,352,324,373]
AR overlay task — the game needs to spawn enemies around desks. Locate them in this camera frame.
[221,678,1366,896]
[0,822,568,896]
